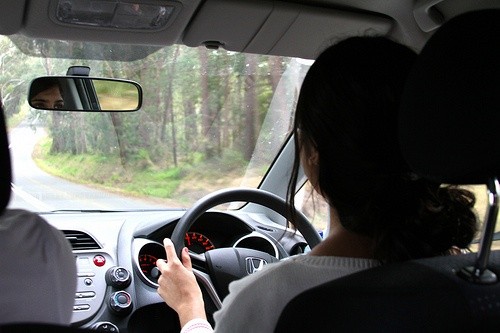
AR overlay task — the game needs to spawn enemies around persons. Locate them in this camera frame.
[156,36,477,333]
[30,78,64,109]
[0,90,77,326]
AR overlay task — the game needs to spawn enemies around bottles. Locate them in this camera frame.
[304,231,325,256]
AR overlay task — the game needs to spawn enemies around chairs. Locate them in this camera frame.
[274,7,500,333]
